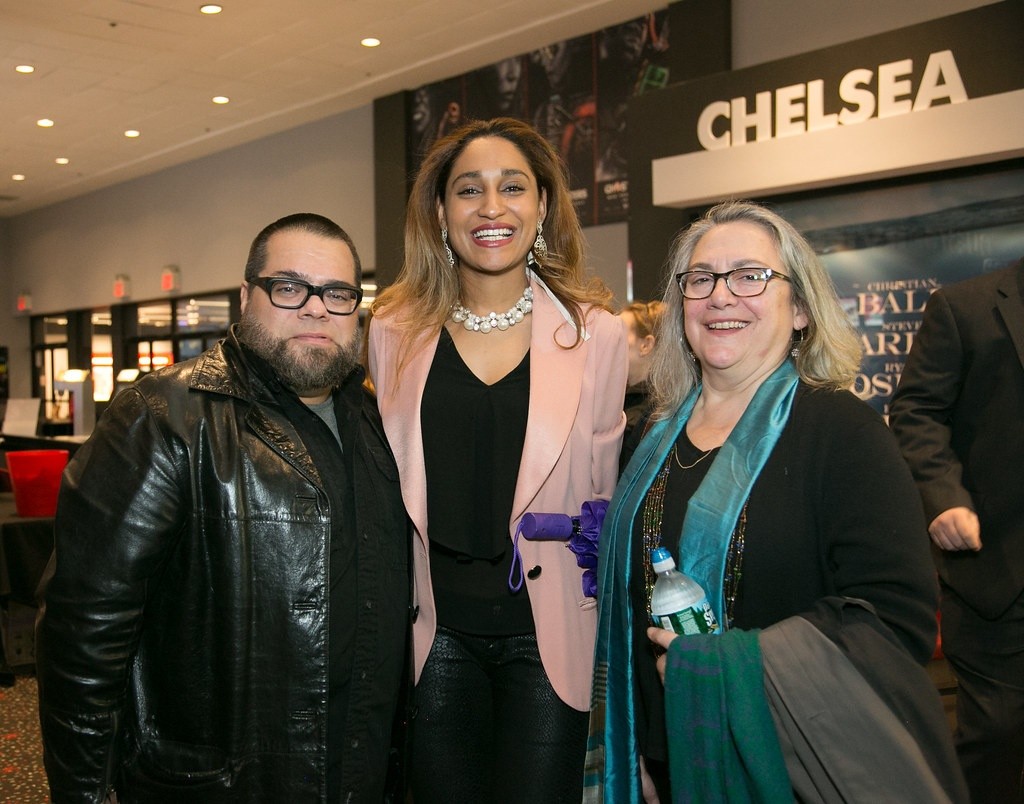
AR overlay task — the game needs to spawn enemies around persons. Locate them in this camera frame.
[581,197,970,804]
[364,117,672,804]
[37,213,414,804]
[889,258,1024,804]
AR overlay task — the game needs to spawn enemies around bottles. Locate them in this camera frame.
[650,549,721,636]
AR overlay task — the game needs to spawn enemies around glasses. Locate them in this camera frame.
[247,276,364,316]
[676,268,792,300]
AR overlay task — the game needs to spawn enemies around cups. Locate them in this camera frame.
[6,450,68,519]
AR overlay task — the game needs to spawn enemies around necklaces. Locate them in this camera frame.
[439,282,533,334]
